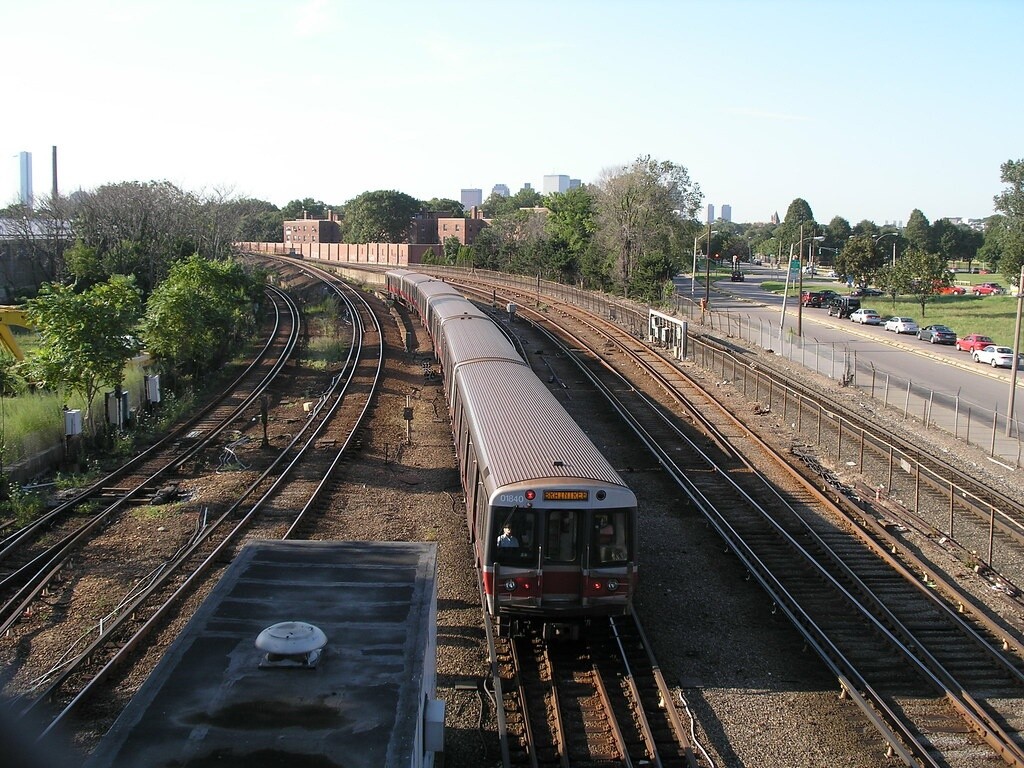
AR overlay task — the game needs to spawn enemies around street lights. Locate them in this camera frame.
[872,232,897,250]
[779,236,825,333]
[691,231,717,294]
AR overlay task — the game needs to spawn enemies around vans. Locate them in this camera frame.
[828,297,860,319]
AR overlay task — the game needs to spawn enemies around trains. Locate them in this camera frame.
[384,268,639,644]
[80,539,446,768]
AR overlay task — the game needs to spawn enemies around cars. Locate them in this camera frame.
[980,269,990,274]
[849,309,881,325]
[956,335,996,355]
[851,289,864,296]
[940,284,966,296]
[916,324,957,345]
[884,317,919,335]
[868,289,884,296]
[972,345,1021,368]
[810,269,817,275]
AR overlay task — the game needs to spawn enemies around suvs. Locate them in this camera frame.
[971,267,980,273]
[819,290,841,300]
[802,292,822,308]
[731,271,744,282]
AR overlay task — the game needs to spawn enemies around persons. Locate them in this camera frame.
[497,522,519,547]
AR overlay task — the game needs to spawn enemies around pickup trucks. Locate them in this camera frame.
[973,282,1005,296]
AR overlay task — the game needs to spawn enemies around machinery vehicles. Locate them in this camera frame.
[0,305,39,361]
